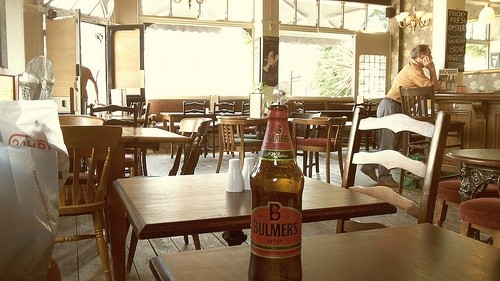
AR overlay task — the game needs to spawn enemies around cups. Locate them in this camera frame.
[240,157,258,191]
[225,159,244,192]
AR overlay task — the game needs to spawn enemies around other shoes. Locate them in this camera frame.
[377,174,399,187]
[360,164,377,183]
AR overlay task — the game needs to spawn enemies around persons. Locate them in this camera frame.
[360,44,439,188]
[81,65,99,115]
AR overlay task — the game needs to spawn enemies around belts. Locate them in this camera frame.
[385,97,401,106]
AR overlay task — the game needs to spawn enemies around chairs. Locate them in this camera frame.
[49,86,500,281]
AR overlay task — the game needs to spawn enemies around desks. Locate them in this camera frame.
[446,149,500,239]
[306,110,378,148]
[112,169,396,247]
[68,126,191,281]
[150,223,500,281]
[160,112,218,159]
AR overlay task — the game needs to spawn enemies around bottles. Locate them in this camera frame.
[248,102,306,280]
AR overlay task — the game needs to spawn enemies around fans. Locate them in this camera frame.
[273,82,291,105]
[18,57,55,101]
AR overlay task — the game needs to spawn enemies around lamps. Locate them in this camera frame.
[479,0,495,23]
[395,0,433,32]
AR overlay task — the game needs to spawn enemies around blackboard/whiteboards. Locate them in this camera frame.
[445,9,468,73]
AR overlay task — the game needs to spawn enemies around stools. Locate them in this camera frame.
[437,180,500,241]
[461,198,500,248]
